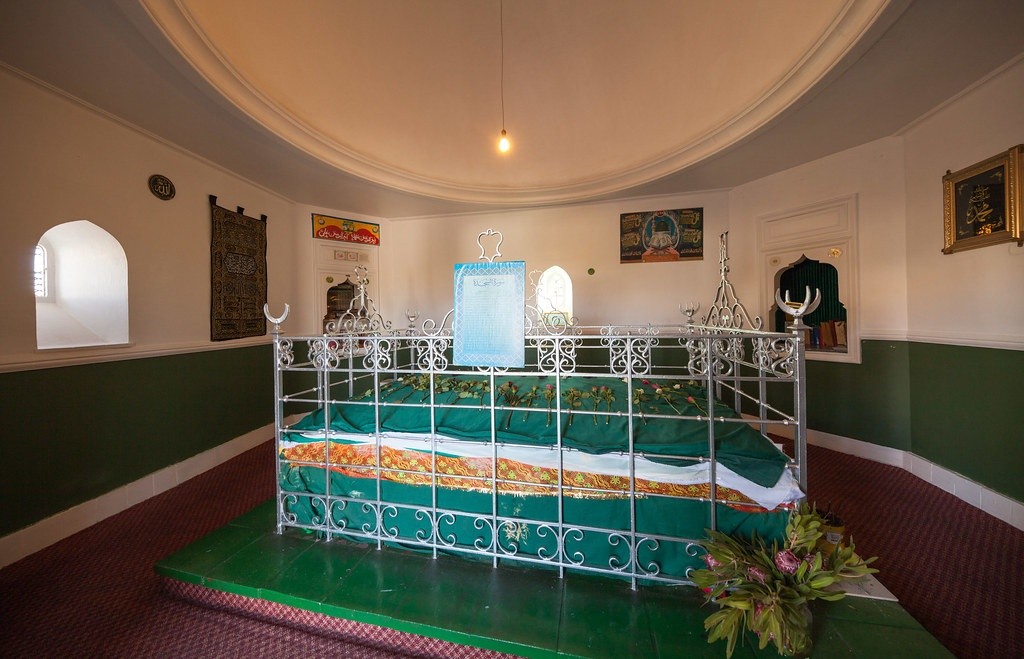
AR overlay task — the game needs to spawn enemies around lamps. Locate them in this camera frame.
[498,0,510,153]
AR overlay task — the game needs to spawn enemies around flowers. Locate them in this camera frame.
[688,501,881,659]
[590,386,604,425]
[542,384,556,427]
[620,377,729,427]
[396,374,415,382]
[450,380,479,406]
[381,375,417,400]
[494,381,513,405]
[562,387,590,426]
[397,373,430,405]
[523,384,541,422]
[476,380,491,409]
[506,392,530,429]
[348,383,392,402]
[502,385,518,404]
[600,385,616,425]
[420,375,458,404]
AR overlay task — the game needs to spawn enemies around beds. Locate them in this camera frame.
[262,231,824,599]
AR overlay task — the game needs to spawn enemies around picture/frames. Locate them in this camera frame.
[941,143,1024,256]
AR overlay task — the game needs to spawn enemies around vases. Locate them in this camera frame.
[781,605,814,649]
